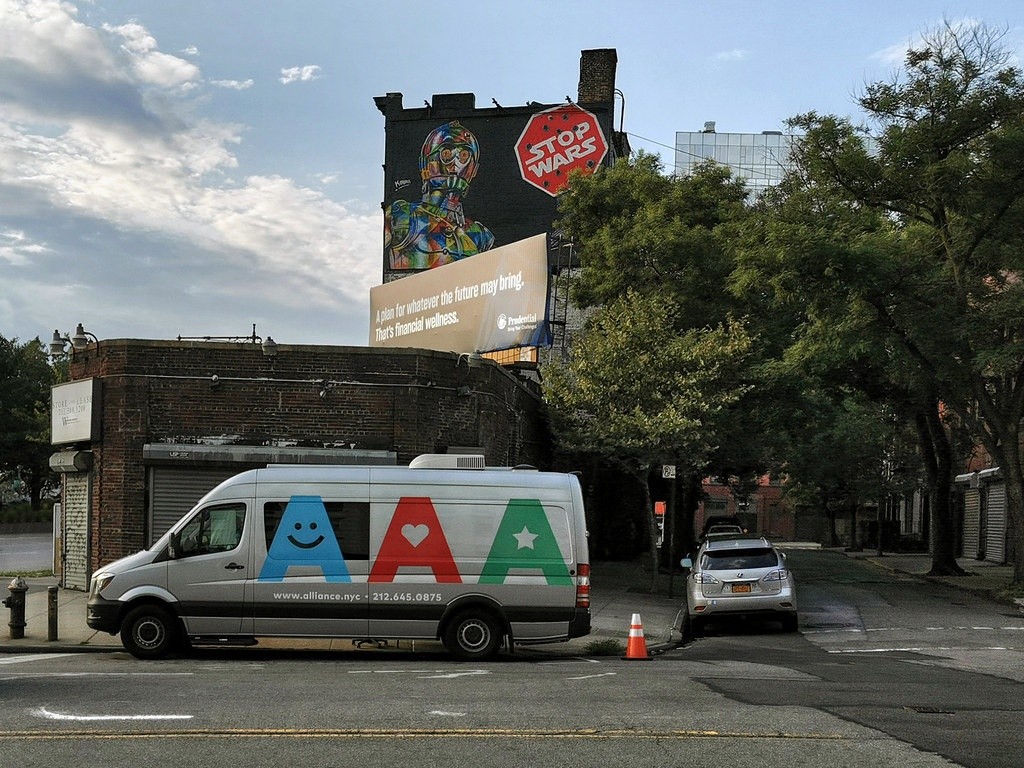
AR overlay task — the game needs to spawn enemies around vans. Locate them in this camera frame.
[85,453,593,662]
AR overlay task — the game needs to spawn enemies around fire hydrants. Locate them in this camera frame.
[1,574,30,639]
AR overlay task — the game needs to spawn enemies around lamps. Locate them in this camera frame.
[262,337,279,357]
[457,349,483,368]
[73,322,99,357]
[50,329,75,361]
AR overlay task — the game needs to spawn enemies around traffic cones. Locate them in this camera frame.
[620,612,654,662]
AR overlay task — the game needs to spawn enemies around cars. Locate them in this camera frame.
[696,515,748,549]
[680,536,799,634]
